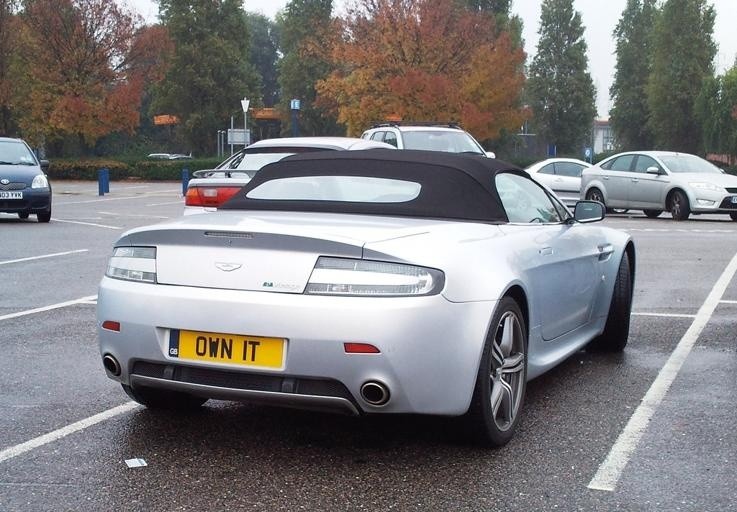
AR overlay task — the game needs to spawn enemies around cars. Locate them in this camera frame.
[0,137,52,222]
[184,136,397,216]
[578,151,737,222]
[524,158,629,214]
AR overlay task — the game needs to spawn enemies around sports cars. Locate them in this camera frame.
[96,147,637,450]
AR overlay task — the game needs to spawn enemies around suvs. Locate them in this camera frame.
[359,121,496,159]
